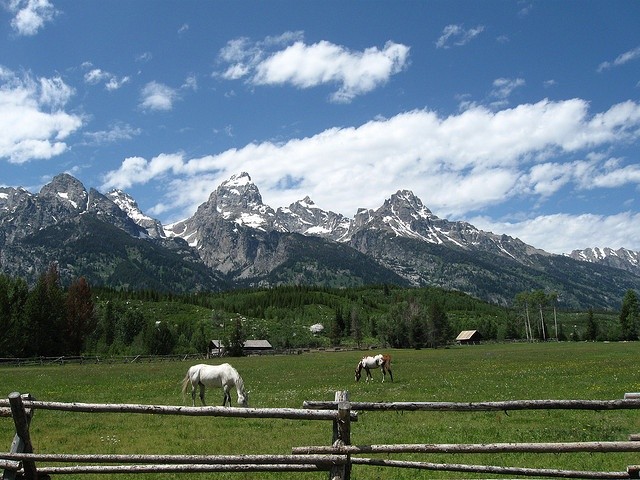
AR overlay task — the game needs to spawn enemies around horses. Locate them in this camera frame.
[181,363,250,407]
[354,353,394,384]
